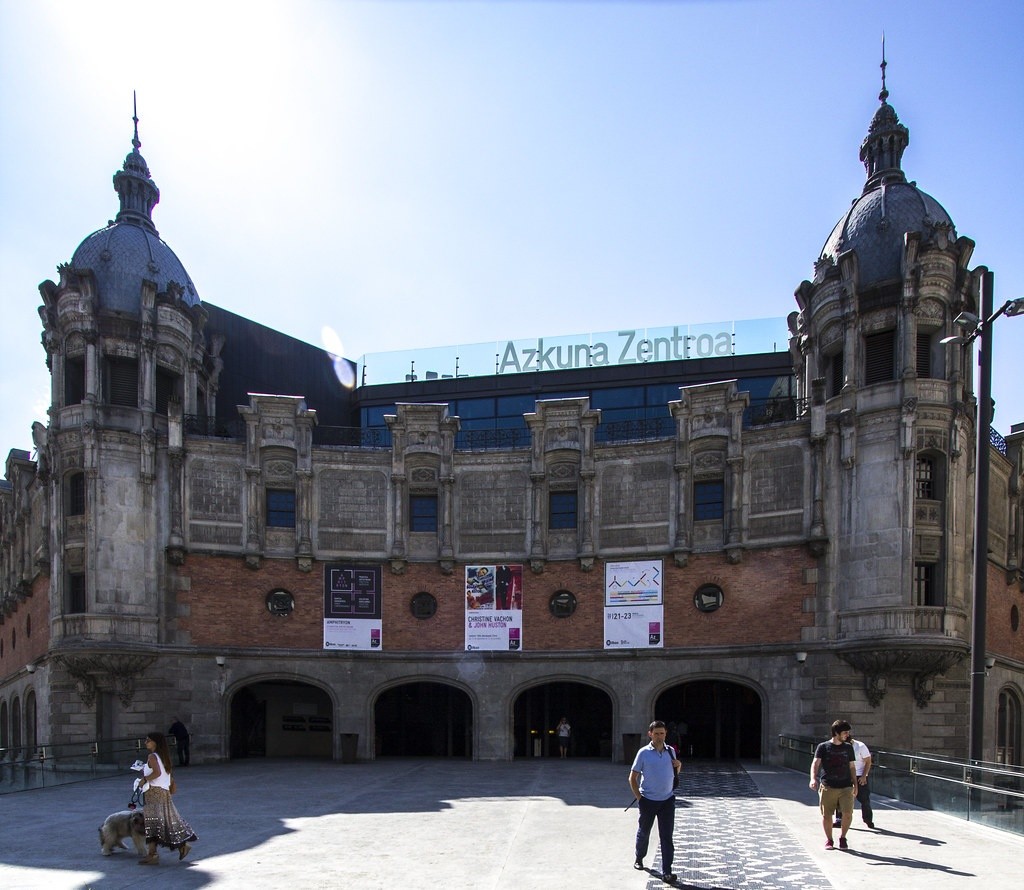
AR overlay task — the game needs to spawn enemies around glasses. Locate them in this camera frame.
[143,739,152,743]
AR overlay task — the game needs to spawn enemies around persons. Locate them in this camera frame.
[467,567,488,585]
[556,717,570,759]
[496,566,511,610]
[169,720,190,767]
[667,722,681,757]
[139,732,197,865]
[809,720,858,850]
[832,735,874,829]
[629,721,682,882]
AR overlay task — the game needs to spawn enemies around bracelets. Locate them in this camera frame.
[144,777,147,782]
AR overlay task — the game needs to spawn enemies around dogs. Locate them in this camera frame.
[98,809,147,856]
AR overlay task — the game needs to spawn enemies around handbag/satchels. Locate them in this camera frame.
[666,745,678,790]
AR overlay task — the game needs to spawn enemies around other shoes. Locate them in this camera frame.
[839,837,848,850]
[662,870,677,882]
[864,820,874,829]
[560,756,564,760]
[180,759,185,764]
[564,755,568,759]
[832,821,841,828]
[634,856,643,870]
[185,763,191,768]
[825,840,833,849]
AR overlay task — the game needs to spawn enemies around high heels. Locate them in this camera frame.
[179,842,192,860]
[138,851,159,865]
